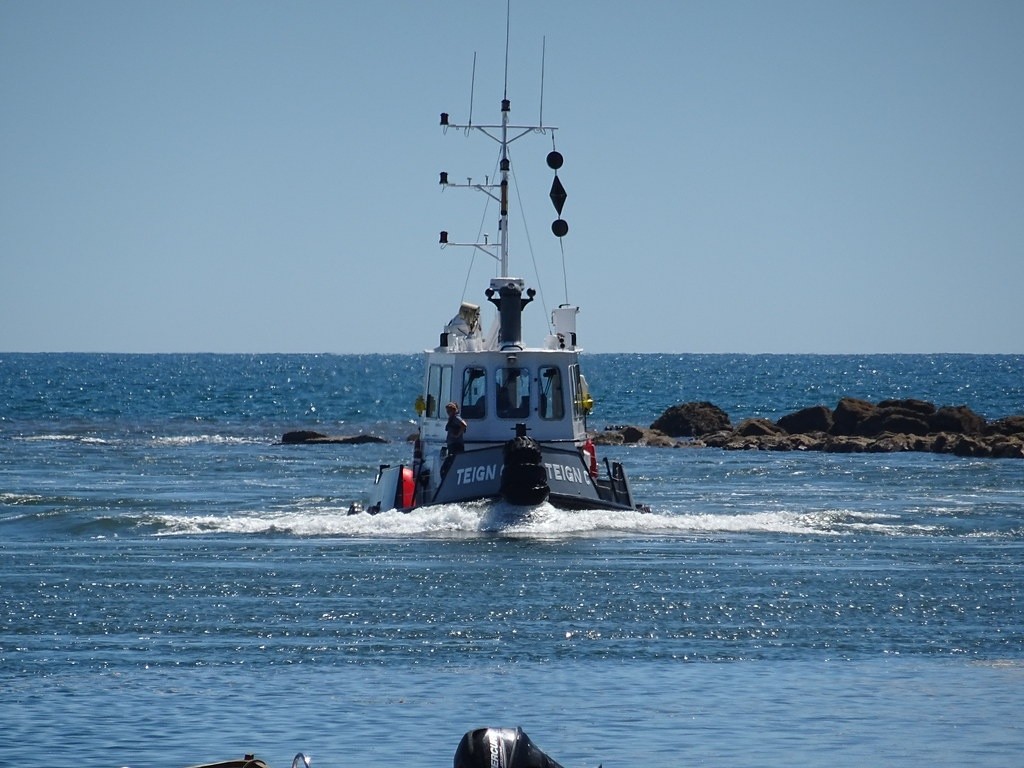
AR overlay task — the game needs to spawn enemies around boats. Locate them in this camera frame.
[362,0,654,514]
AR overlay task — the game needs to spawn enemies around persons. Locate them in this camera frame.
[445,403,469,453]
